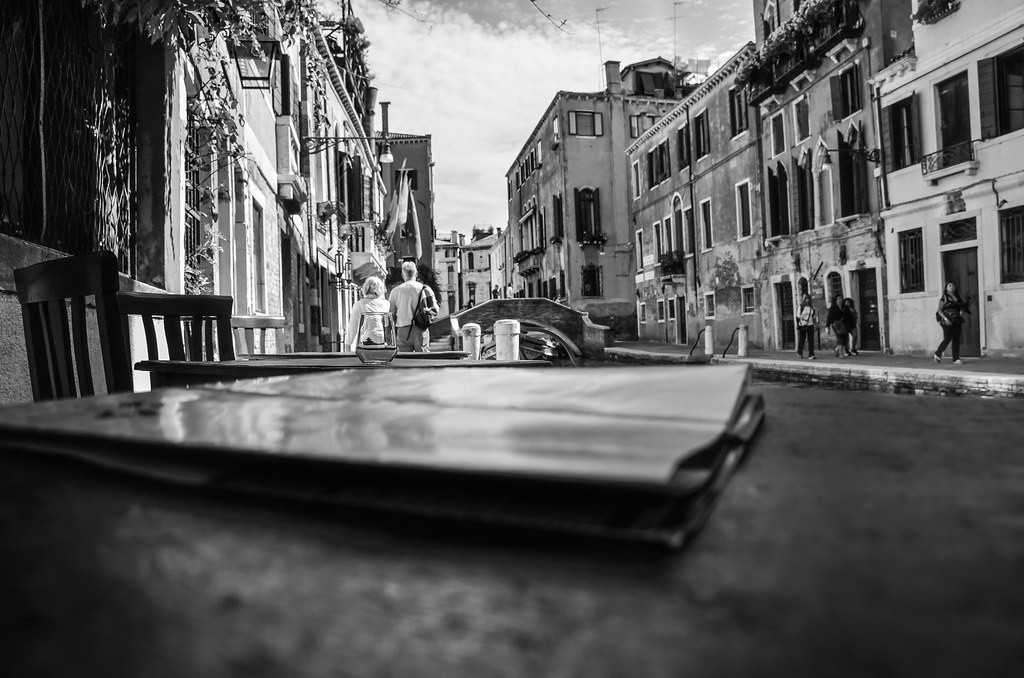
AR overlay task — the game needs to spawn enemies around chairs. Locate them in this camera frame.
[14,250,134,402]
[119,289,234,396]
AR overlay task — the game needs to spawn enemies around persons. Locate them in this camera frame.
[825,295,858,357]
[468,294,475,308]
[795,294,817,360]
[492,285,500,298]
[506,283,514,298]
[346,277,390,353]
[932,282,968,365]
[387,261,440,352]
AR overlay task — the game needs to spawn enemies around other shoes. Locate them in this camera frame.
[809,356,816,359]
[840,353,845,359]
[953,358,962,365]
[934,354,941,363]
[851,350,858,355]
[835,348,839,356]
[798,353,803,359]
[844,352,851,356]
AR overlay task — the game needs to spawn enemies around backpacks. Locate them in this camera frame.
[414,286,437,331]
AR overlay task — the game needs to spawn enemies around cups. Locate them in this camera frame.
[355,311,397,364]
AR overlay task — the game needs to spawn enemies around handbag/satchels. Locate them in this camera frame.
[833,320,846,335]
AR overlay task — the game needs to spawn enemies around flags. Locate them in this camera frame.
[386,174,422,260]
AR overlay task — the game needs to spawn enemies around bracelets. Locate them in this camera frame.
[944,317,948,321]
